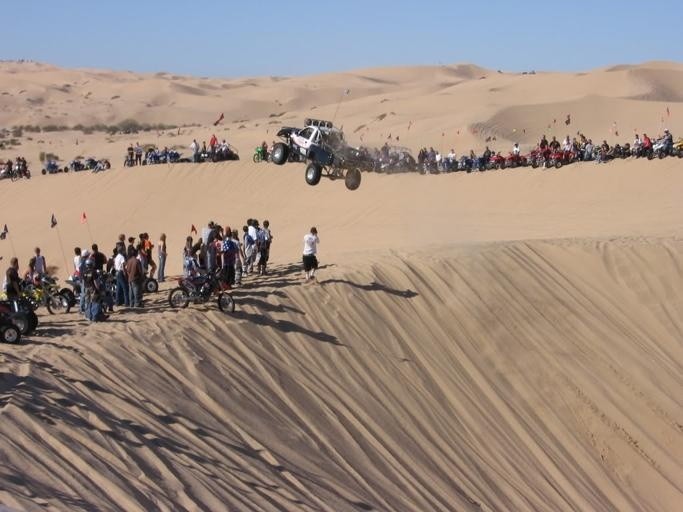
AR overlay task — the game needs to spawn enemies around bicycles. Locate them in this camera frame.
[122,155,135,168]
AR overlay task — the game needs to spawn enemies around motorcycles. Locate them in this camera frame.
[167,266,236,314]
[361,149,528,174]
[0,272,158,344]
[529,142,613,170]
[0,164,30,181]
[615,136,683,160]
[252,147,270,163]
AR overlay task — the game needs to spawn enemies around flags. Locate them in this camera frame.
[213,112,224,126]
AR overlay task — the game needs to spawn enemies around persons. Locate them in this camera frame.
[532,134,609,170]
[373,142,391,172]
[630,129,672,158]
[469,141,520,172]
[302,227,321,282]
[4,155,27,179]
[147,146,171,165]
[0,214,272,341]
[127,141,142,166]
[418,146,456,175]
[262,139,275,161]
[400,152,410,163]
[50,156,95,173]
[169,147,183,157]
[190,133,228,162]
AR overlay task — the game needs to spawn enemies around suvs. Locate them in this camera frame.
[198,144,239,162]
[271,119,363,191]
